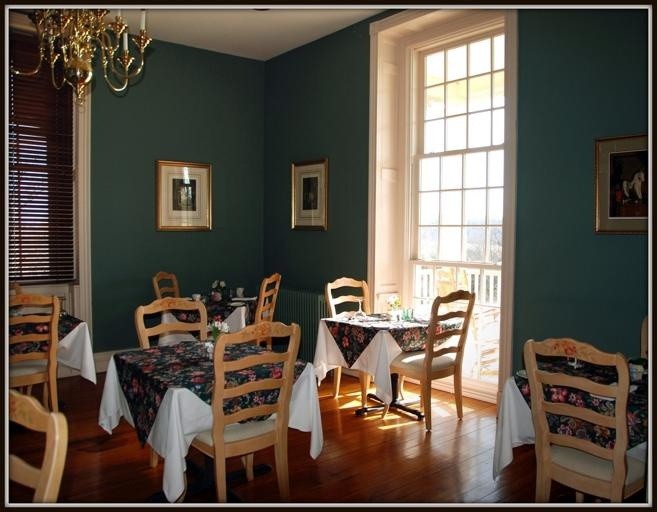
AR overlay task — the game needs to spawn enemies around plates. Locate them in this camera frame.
[232,298,257,300]
[346,312,392,322]
[228,302,245,306]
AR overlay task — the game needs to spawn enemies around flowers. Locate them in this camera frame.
[211,320,230,342]
[208,280,226,302]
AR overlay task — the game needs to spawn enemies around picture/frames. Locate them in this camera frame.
[156,160,212,233]
[595,135,649,235]
[290,158,330,232]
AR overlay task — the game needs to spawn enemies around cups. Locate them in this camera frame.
[236,288,243,297]
[192,294,201,301]
[630,364,645,381]
[354,297,366,320]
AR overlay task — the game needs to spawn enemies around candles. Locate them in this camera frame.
[122,33,128,51]
[117,9,122,16]
[140,10,146,31]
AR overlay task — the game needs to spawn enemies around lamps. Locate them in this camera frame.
[8,9,152,106]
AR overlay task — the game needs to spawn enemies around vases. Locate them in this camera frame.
[387,310,401,321]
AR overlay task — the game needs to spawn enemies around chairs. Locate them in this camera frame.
[325,277,372,408]
[153,271,179,300]
[9,292,61,414]
[248,273,282,351]
[9,391,69,504]
[134,296,206,348]
[380,290,475,429]
[190,320,300,502]
[524,337,645,504]
